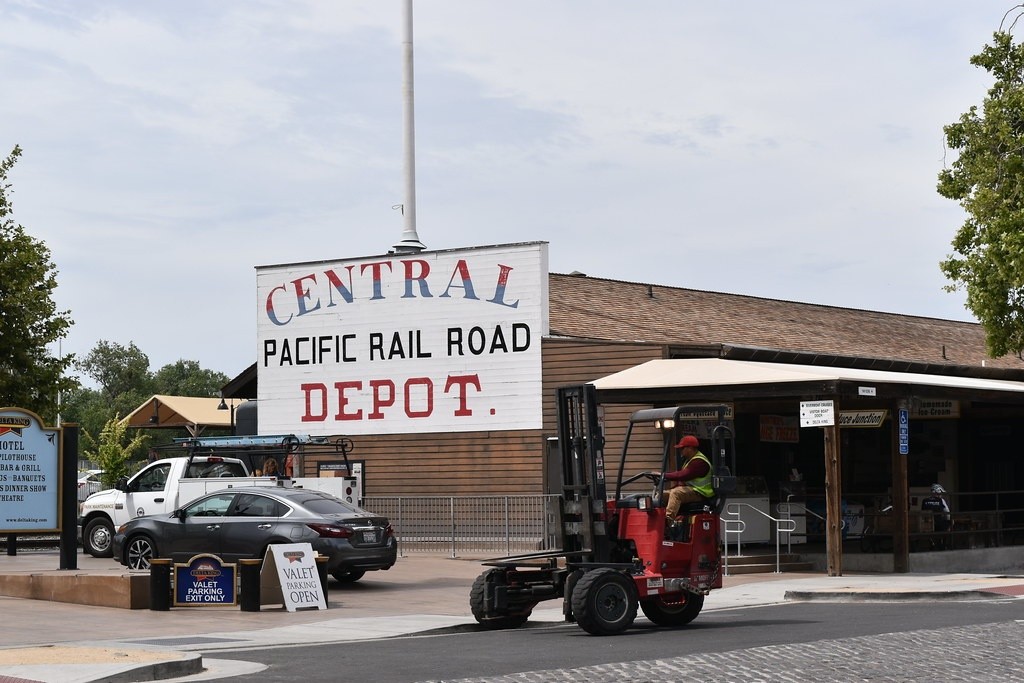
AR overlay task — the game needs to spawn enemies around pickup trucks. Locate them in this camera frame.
[79,434,359,559]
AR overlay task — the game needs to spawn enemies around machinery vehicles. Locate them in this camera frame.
[467,382,738,634]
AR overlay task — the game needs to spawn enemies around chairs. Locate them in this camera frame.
[676,465,732,514]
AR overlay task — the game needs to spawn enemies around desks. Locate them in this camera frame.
[951,519,986,550]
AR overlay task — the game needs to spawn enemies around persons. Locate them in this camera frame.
[921,484,954,525]
[652,436,710,526]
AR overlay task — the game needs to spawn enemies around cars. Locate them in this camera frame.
[112,487,398,585]
[78,470,129,503]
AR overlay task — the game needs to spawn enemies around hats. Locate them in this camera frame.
[931,484,946,493]
[674,435,699,448]
